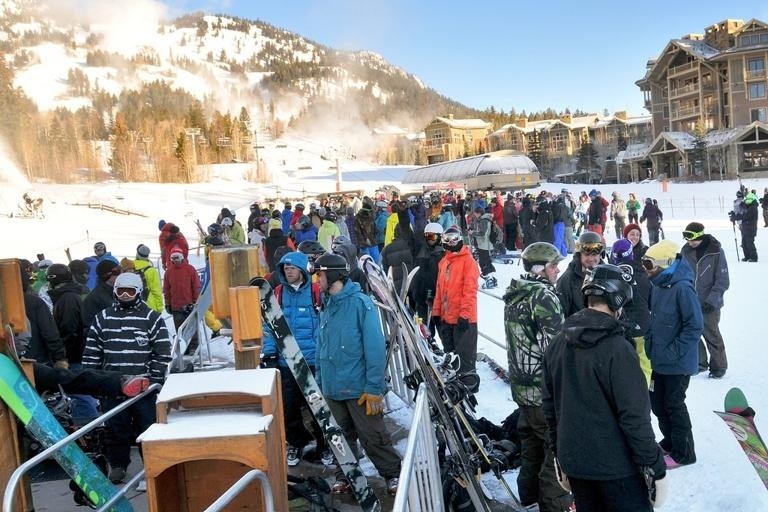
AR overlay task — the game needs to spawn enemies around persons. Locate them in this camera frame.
[1,175,768,512]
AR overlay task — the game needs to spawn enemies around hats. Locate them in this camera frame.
[284,263,300,271]
[587,189,597,197]
[613,240,633,264]
[624,224,641,239]
[158,220,165,230]
[137,246,150,259]
[645,240,679,269]
[686,223,704,241]
[170,252,185,261]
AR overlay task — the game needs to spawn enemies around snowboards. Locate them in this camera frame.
[175,266,210,355]
[0,326,138,512]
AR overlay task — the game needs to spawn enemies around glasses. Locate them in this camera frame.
[116,287,138,297]
[171,256,181,260]
[578,243,602,254]
[682,230,704,240]
[442,234,460,243]
[640,256,675,271]
[424,232,439,241]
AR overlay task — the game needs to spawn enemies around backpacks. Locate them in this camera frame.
[484,218,503,244]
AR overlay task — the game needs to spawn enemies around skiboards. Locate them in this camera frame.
[713,388,768,491]
[357,253,532,512]
[367,260,532,512]
[249,276,382,511]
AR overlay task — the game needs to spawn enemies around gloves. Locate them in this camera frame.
[165,304,172,314]
[457,317,469,331]
[261,358,282,367]
[358,394,385,415]
[432,316,440,323]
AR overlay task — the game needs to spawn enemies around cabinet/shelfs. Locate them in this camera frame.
[136,368,289,512]
[0,399,27,511]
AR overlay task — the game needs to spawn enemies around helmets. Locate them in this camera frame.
[324,212,339,222]
[576,233,602,255]
[93,242,106,254]
[521,242,565,274]
[208,201,310,235]
[112,272,144,296]
[314,254,348,282]
[424,223,443,234]
[297,241,325,263]
[21,259,90,288]
[444,223,462,248]
[170,247,183,255]
[582,264,634,312]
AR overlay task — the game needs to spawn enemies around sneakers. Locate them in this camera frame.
[663,455,682,468]
[482,276,497,289]
[697,363,725,379]
[287,445,302,466]
[386,477,397,494]
[120,375,149,397]
[109,468,126,482]
[332,481,351,491]
[319,447,333,466]
